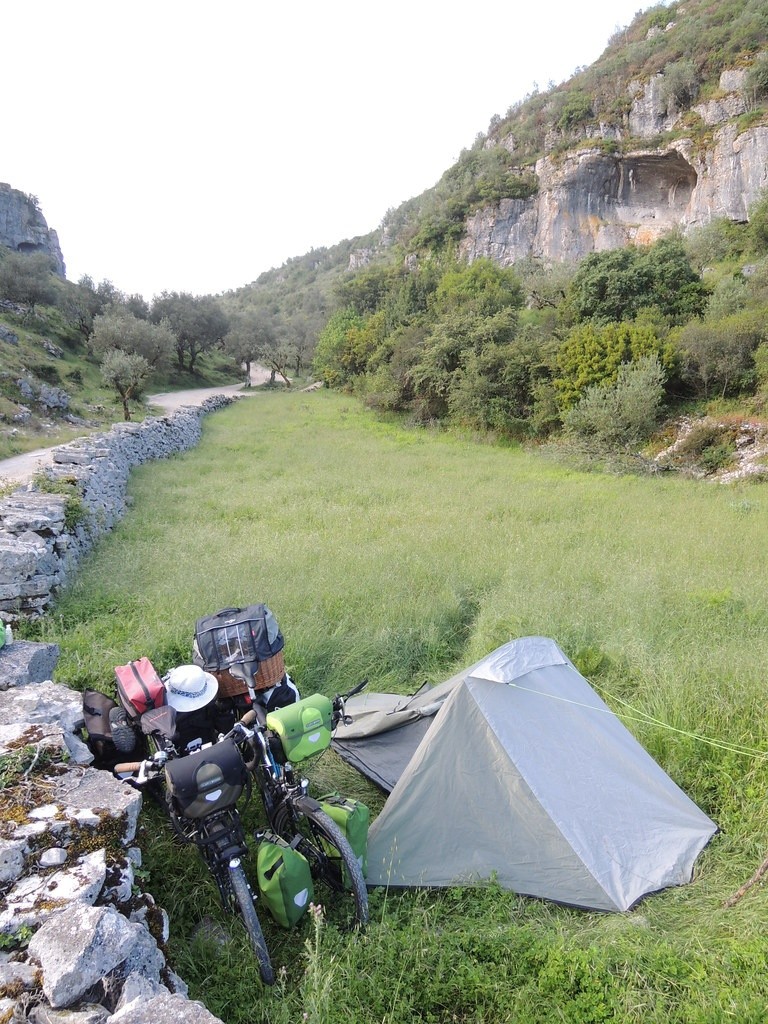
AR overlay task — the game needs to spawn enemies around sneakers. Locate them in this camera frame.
[109,706,135,753]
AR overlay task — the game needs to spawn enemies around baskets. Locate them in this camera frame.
[208,648,285,698]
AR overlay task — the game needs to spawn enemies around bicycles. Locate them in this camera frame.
[113,666,373,987]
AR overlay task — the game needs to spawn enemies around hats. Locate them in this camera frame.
[164,664,218,712]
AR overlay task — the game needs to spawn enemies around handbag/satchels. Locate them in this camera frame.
[83,687,120,758]
[255,829,314,930]
[193,603,285,671]
[166,739,253,844]
[266,693,333,763]
[115,656,166,721]
[306,791,368,890]
